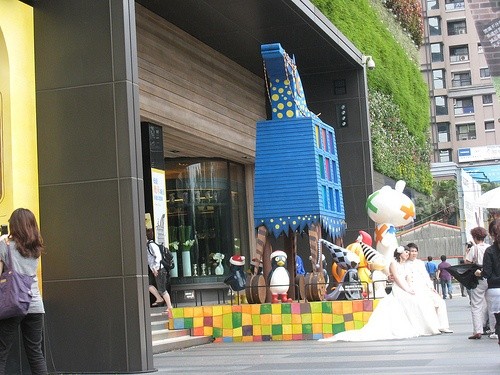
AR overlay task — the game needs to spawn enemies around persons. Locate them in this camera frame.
[388,243,453,335]
[438,255,452,299]
[0,208,48,375]
[425,256,439,294]
[146,228,173,312]
[460,219,500,344]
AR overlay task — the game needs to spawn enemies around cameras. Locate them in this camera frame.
[468,244,472,248]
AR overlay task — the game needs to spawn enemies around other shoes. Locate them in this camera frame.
[469,334,482,340]
[489,332,498,338]
[439,328,453,333]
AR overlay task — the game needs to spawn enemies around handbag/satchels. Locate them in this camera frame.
[148,241,175,271]
[0,269,36,320]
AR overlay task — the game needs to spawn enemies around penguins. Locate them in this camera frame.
[267,255,300,304]
[223,262,250,295]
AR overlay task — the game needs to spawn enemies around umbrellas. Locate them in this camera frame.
[441,263,483,290]
